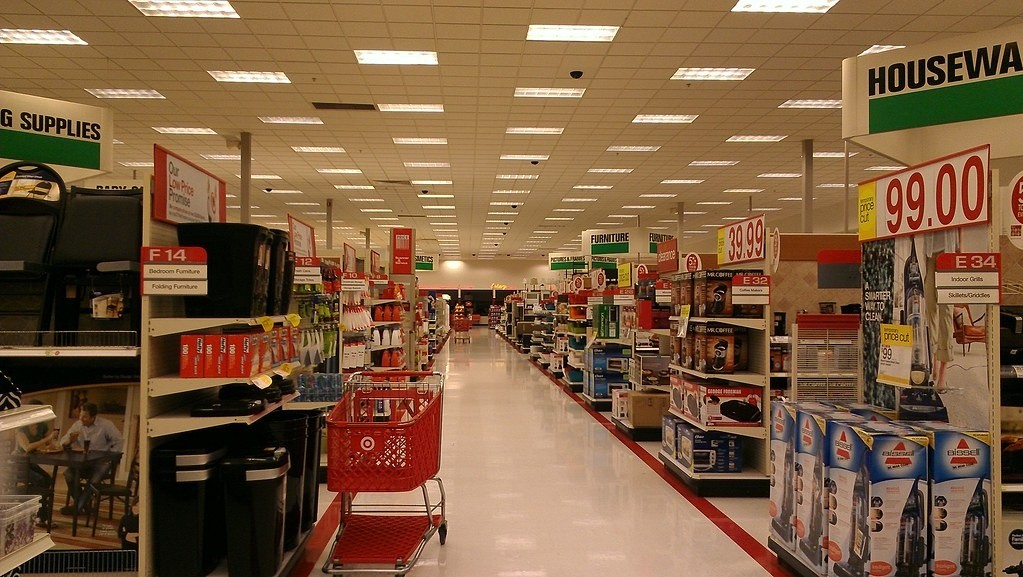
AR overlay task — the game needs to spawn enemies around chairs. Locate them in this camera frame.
[1,438,140,536]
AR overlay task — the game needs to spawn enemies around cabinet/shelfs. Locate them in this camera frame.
[767,22,1023,577]
[0,173,314,577]
[370,281,409,372]
[494,263,676,441]
[657,231,863,496]
[293,274,375,391]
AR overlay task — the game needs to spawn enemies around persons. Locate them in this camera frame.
[922,228,963,393]
[14,400,123,528]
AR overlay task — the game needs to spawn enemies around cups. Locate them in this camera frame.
[53,425,60,441]
[774,311,786,335]
[83,436,90,455]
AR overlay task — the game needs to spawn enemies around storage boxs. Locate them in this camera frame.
[507,271,1023,577]
[178,326,301,380]
[413,296,450,372]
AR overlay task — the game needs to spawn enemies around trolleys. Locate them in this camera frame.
[316,369,448,575]
[452,315,472,344]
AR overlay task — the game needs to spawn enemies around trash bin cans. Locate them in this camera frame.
[152,410,323,577]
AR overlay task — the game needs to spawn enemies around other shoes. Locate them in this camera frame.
[61,506,78,515]
[36,519,47,528]
[42,519,58,529]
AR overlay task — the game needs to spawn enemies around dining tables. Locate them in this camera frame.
[11,450,122,536]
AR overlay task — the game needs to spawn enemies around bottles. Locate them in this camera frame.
[374,303,406,367]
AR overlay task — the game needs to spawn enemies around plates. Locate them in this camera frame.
[41,449,64,454]
[69,448,86,452]
